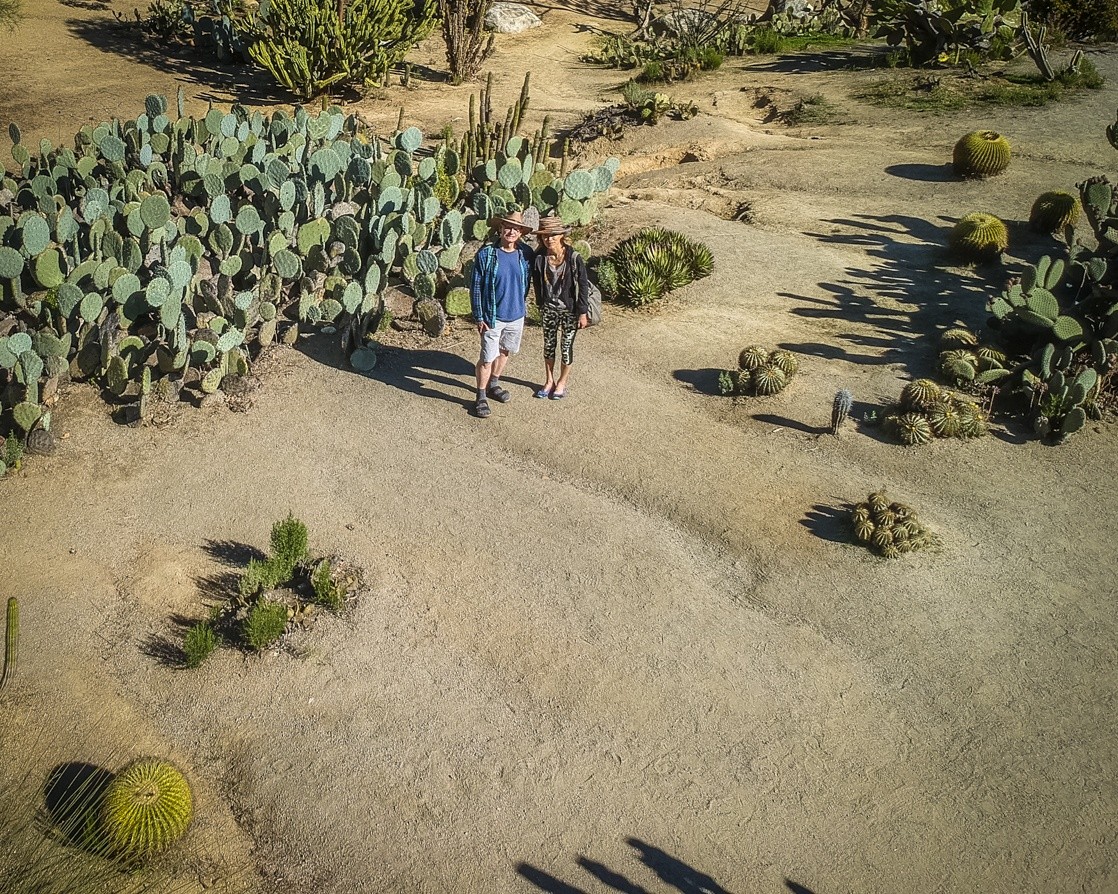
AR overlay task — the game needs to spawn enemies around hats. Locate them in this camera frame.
[530,217,572,234]
[485,211,533,237]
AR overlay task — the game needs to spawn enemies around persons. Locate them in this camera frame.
[470,210,535,417]
[533,217,589,400]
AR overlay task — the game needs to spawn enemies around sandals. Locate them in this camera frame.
[475,399,492,418]
[486,386,510,403]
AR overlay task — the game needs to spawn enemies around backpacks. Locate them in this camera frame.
[571,251,602,328]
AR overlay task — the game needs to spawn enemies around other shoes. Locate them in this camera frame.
[553,385,565,400]
[536,381,555,398]
